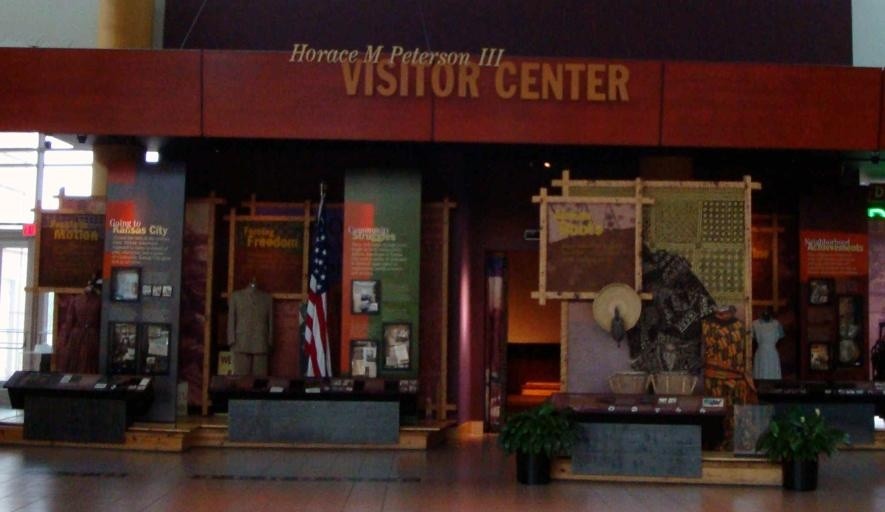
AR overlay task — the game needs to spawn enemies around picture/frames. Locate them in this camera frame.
[109,265,140,302]
[835,291,864,370]
[380,320,415,372]
[352,277,382,316]
[807,273,835,308]
[348,338,382,382]
[138,319,172,378]
[807,341,831,374]
[109,322,135,377]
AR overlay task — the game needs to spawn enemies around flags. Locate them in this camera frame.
[484,250,506,426]
[297,192,334,378]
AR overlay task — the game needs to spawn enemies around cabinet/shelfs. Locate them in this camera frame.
[211,391,416,449]
[751,378,885,450]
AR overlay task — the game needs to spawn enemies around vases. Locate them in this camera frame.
[778,446,821,493]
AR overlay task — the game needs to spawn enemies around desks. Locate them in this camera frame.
[2,369,153,444]
[537,392,731,479]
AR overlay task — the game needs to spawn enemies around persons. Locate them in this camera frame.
[225,281,275,378]
[179,232,207,366]
[871,320,885,383]
[61,279,100,375]
[751,308,784,380]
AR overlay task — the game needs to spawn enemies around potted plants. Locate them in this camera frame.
[502,402,577,487]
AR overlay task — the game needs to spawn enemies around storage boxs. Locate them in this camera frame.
[216,352,252,378]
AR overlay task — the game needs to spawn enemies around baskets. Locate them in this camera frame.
[652,371,698,396]
[610,368,650,396]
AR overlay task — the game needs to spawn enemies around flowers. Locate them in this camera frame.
[752,407,847,464]
